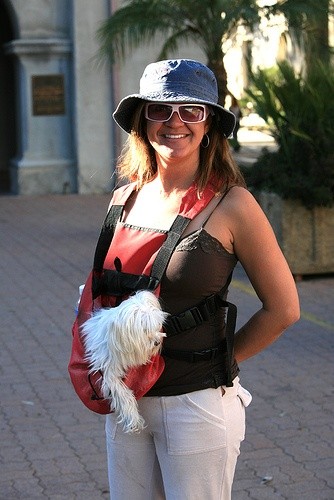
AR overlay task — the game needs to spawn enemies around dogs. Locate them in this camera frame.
[81,288,168,432]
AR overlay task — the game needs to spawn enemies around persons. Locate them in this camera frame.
[94,60,300,500]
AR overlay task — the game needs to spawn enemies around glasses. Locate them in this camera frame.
[143,102,211,123]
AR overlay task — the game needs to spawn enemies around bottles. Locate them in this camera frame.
[74,284,85,316]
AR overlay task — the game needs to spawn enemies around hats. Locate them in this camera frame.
[112,59,237,140]
